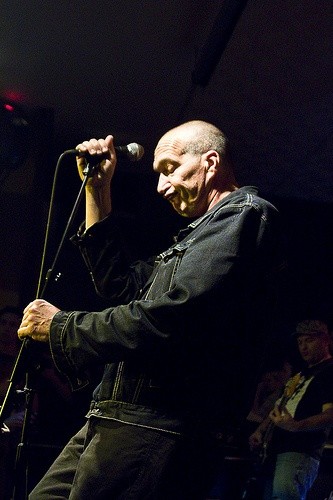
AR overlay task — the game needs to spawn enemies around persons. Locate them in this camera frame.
[245,319,333,499]
[0,307,38,458]
[238,357,294,450]
[42,346,92,405]
[15,119,286,500]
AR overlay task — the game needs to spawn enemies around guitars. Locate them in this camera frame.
[246,371,306,464]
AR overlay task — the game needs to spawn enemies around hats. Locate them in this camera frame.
[295,320,328,338]
